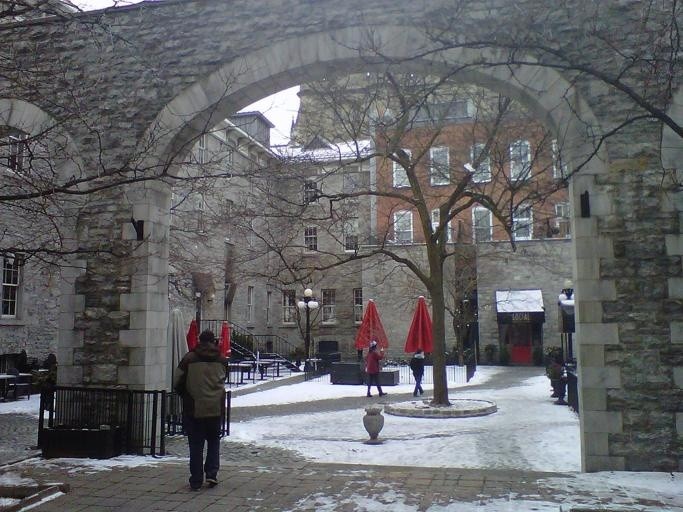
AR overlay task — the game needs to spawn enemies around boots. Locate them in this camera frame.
[367,388,387,397]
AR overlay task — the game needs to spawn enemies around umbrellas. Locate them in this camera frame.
[404,295,432,352]
[355,299,389,349]
[169,309,189,391]
[218,320,231,358]
[187,318,199,351]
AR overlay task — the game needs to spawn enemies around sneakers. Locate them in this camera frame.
[191,477,220,491]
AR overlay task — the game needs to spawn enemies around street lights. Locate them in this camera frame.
[557,279,575,367]
[296,287,320,372]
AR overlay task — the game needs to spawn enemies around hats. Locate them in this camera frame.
[199,329,214,343]
[370,341,377,347]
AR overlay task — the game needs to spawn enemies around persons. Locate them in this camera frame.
[173,329,228,491]
[365,341,387,397]
[411,350,425,396]
